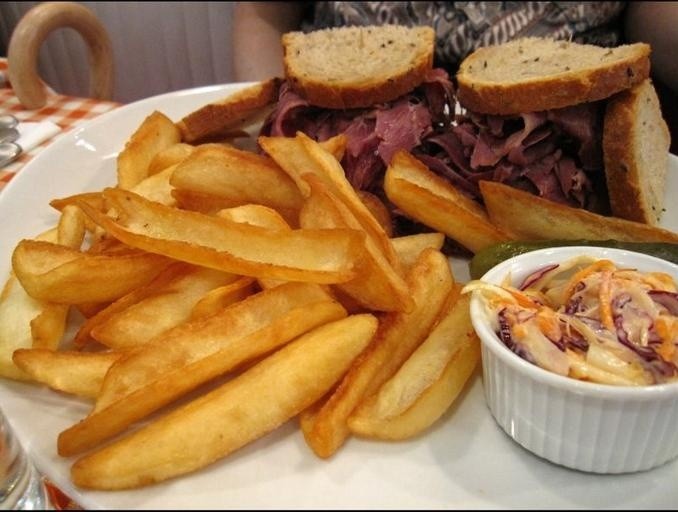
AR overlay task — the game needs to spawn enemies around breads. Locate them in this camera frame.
[456,36,653,117]
[173,77,285,144]
[281,24,435,109]
[601,79,671,225]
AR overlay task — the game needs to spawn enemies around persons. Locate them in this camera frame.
[230,0,677,154]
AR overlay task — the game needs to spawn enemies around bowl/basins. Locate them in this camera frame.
[467,247,677,475]
